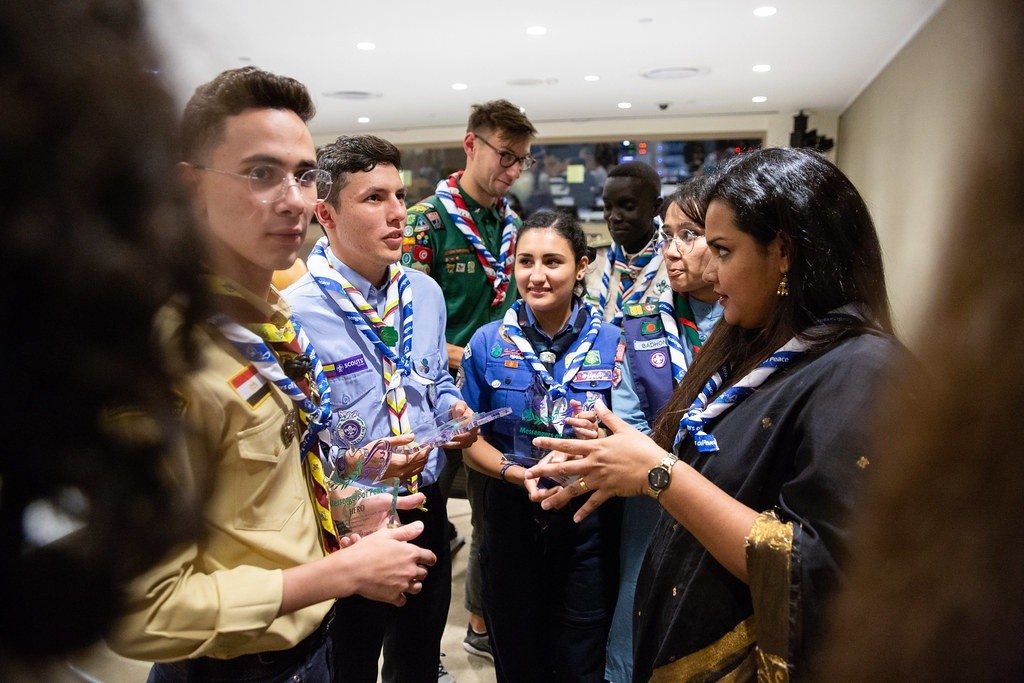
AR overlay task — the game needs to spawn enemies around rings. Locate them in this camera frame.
[579,478,590,494]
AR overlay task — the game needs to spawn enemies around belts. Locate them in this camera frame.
[271,605,342,670]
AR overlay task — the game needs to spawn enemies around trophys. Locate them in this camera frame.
[511,397,592,487]
[325,436,396,537]
[397,408,513,454]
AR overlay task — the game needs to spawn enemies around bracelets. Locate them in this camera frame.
[499,460,517,481]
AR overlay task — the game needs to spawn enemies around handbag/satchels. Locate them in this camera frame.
[647,507,794,683]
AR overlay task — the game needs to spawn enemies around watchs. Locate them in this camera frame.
[648,454,679,498]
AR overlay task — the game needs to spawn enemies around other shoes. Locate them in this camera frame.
[437,652,456,683]
[449,528,465,555]
[462,621,494,664]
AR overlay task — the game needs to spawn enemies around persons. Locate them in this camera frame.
[0,1,219,683]
[823,6,1023,683]
[417,144,739,219]
[281,134,479,683]
[521,147,919,683]
[93,65,436,683]
[400,100,731,663]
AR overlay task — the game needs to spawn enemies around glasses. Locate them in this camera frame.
[474,134,536,171]
[190,164,334,206]
[652,228,702,256]
